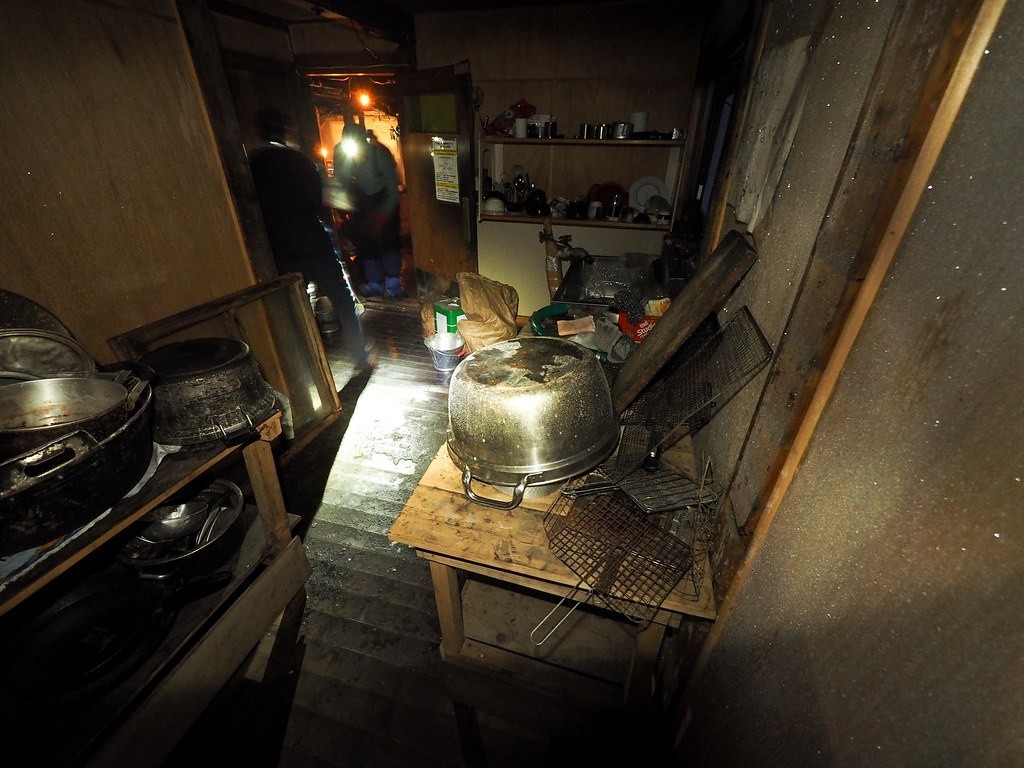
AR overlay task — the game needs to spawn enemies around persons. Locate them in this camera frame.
[331,125,408,298]
[247,107,377,371]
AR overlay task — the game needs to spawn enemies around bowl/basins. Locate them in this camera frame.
[485,199,505,216]
[139,502,209,540]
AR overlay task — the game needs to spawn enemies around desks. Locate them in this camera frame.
[0,408,313,768]
[388,311,716,715]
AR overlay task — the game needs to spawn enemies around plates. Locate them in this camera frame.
[629,175,665,214]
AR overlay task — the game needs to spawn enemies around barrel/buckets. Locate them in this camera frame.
[432,297,466,334]
[425,336,466,372]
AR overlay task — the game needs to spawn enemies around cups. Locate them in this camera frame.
[597,124,610,139]
[613,122,631,139]
[580,124,591,140]
[513,118,527,138]
[531,127,544,139]
[632,111,647,131]
[545,121,556,138]
[656,209,670,224]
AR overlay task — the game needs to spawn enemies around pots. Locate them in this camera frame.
[447,336,619,506]
[0,281,272,587]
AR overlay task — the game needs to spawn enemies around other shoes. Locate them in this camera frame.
[359,284,374,298]
[388,291,408,301]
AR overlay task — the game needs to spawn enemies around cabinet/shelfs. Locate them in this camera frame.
[477,128,689,328]
